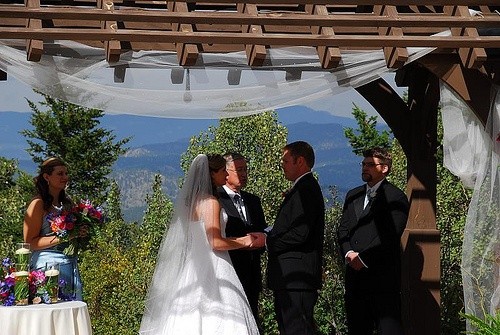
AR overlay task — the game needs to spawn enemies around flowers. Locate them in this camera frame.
[47,198,110,255]
[0,258,77,307]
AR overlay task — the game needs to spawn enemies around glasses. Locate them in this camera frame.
[359,162,386,168]
[227,168,248,173]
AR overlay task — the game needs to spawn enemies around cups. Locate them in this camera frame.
[13,243,31,306]
[45,261,59,304]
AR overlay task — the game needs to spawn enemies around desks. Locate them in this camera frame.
[0,300,92,335]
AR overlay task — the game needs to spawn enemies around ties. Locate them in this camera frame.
[234,194,245,222]
[363,189,371,210]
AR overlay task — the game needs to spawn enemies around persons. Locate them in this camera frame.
[140,152,256,335]
[338,145,407,335]
[247,141,325,335]
[217,152,267,302]
[22,156,86,302]
